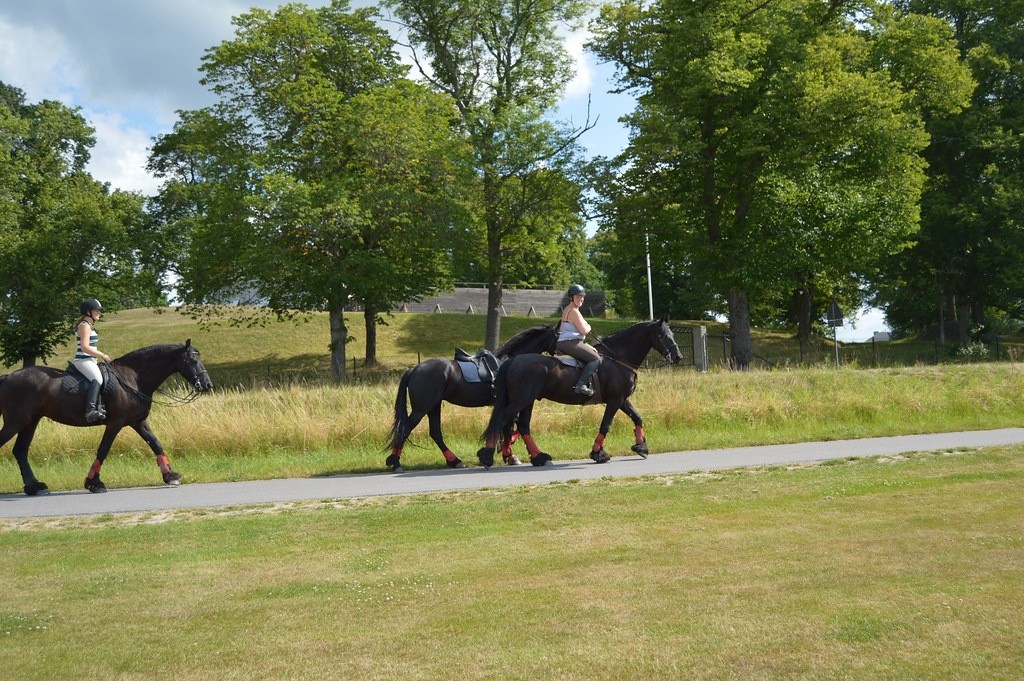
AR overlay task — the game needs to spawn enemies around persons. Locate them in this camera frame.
[556,285,602,397]
[72,298,109,421]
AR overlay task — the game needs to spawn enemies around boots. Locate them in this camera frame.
[85,379,107,422]
[574,359,601,394]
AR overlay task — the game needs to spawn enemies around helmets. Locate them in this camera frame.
[567,284,587,296]
[80,299,102,313]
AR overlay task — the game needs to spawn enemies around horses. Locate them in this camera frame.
[0,339,213,494]
[382,315,684,472]
